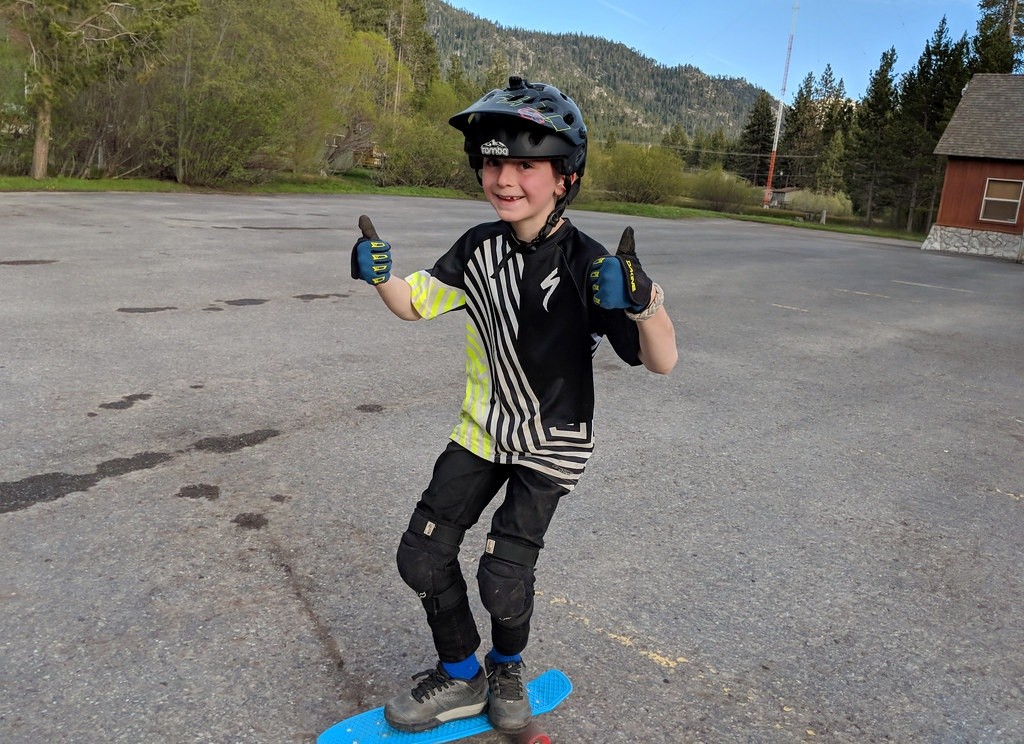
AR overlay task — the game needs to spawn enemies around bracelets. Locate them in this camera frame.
[624,284,664,321]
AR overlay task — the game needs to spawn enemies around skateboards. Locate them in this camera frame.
[315,666,576,744]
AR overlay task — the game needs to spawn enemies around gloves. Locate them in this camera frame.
[590,225,652,314]
[350,215,393,285]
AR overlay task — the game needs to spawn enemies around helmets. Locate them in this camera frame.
[449,76,589,177]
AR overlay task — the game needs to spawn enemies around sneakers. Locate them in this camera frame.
[484,656,533,734]
[384,660,490,732]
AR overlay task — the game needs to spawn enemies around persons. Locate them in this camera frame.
[350,77,678,730]
